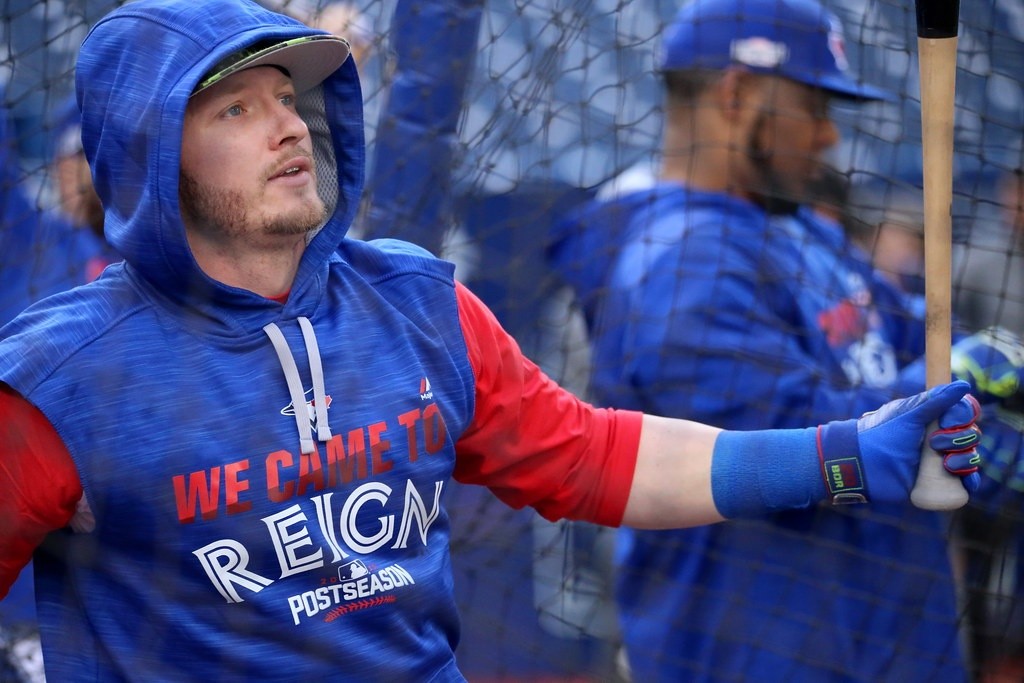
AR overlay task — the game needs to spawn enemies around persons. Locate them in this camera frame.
[0,0,983,683]
[556,0,1023,683]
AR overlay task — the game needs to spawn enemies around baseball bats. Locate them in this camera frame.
[910,0,971,511]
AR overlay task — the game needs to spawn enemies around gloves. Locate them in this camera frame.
[710,382,982,522]
[897,331,1022,399]
[964,401,1024,511]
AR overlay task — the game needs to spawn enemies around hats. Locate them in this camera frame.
[186,33,350,98]
[656,1,901,106]
